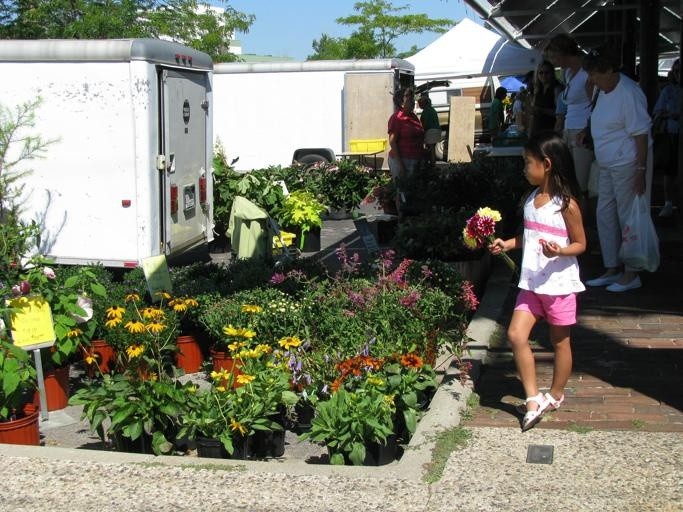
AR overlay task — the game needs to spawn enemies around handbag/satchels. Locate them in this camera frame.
[582,116,595,149]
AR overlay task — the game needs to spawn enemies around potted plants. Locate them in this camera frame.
[316,158,364,220]
[281,187,328,252]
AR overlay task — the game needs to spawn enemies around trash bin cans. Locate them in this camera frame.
[475,126,524,216]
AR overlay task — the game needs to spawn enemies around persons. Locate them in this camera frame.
[418,96,440,167]
[488,131,587,432]
[488,35,683,293]
[388,88,425,215]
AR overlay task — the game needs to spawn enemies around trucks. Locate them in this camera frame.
[0,36,216,268]
[414,76,506,161]
[213,58,416,173]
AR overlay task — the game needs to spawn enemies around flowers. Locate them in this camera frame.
[463,206,521,280]
[1,253,481,456]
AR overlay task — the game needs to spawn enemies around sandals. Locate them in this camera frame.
[516,392,565,432]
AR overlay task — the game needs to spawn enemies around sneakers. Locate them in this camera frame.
[659,205,673,219]
[585,271,624,287]
[606,274,642,292]
